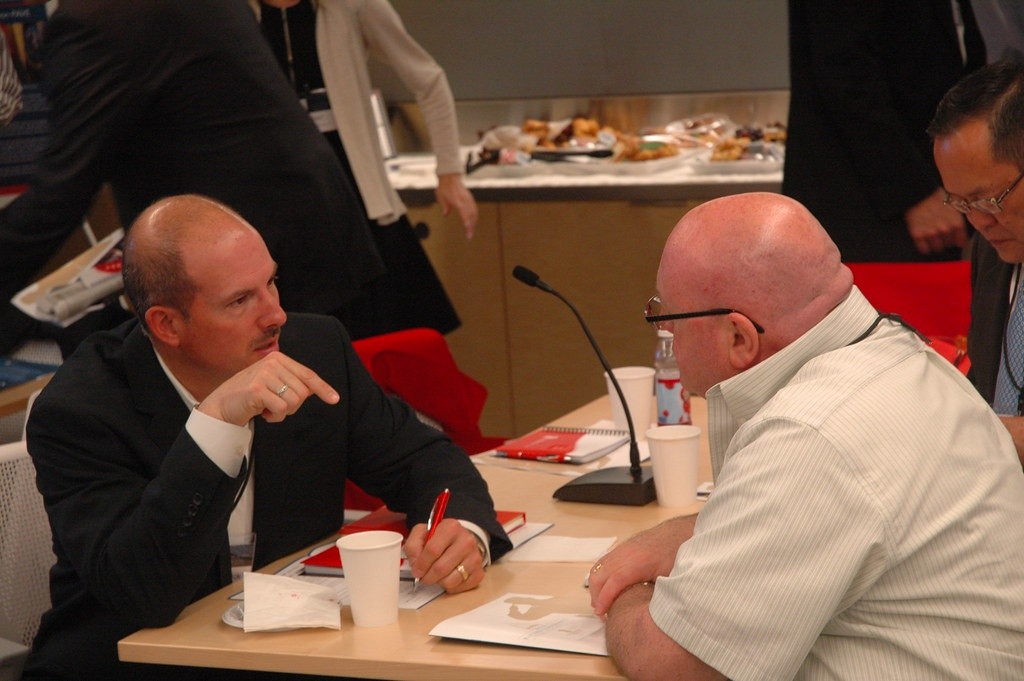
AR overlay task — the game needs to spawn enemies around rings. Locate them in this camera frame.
[593,563,602,573]
[276,384,288,396]
[456,565,470,580]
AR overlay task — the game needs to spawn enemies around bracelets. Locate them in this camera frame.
[468,533,485,558]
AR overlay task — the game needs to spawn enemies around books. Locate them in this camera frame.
[300,507,526,581]
[429,592,607,656]
[490,425,631,465]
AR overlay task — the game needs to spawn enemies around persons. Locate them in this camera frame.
[782,0,1024,468]
[592,191,1024,681]
[0,0,479,340]
[22,196,514,681]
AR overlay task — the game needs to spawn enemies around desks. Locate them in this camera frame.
[117,392,712,680]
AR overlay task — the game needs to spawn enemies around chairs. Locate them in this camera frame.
[0,442,55,681]
[340,327,506,524]
[840,258,972,380]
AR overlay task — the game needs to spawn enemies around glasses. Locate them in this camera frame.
[943,173,1024,216]
[646,295,764,338]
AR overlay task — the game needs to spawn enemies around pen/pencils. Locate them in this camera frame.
[409,488,450,595]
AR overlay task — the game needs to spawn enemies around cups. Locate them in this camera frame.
[647,425,701,509]
[336,531,404,625]
[604,366,657,441]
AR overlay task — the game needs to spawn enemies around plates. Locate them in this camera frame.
[477,126,786,173]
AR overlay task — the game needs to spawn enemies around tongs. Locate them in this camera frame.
[528,146,614,162]
[465,146,498,174]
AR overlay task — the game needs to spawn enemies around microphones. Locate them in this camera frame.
[510,264,656,506]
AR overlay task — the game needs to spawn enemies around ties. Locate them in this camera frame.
[992,279,1024,412]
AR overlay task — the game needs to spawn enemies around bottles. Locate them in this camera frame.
[653,329,692,426]
